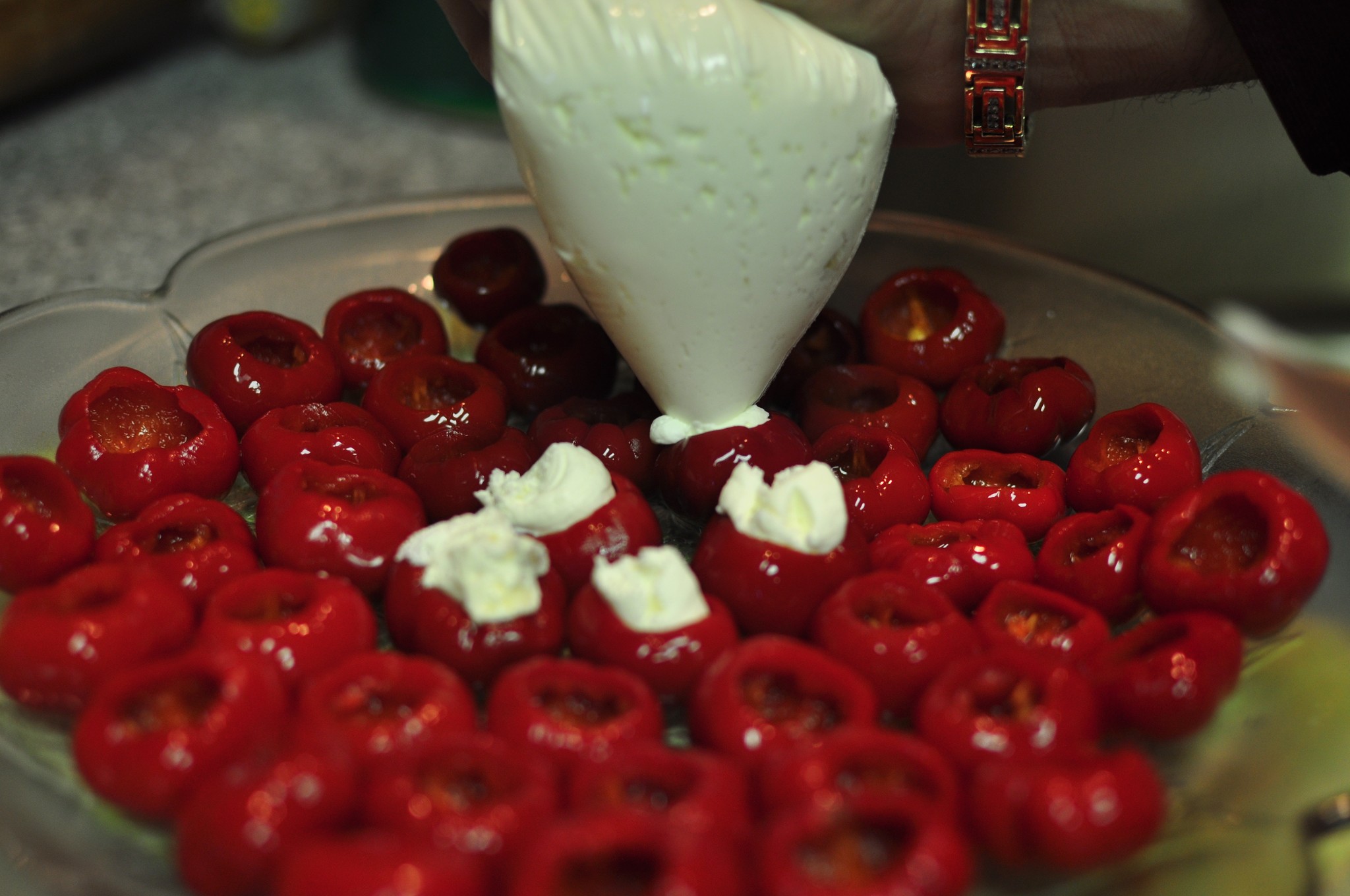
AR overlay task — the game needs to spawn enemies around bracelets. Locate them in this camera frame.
[959,0,1032,159]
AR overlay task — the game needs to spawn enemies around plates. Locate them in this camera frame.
[1,186,1350,896]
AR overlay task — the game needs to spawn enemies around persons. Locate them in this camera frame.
[433,0,1350,192]
